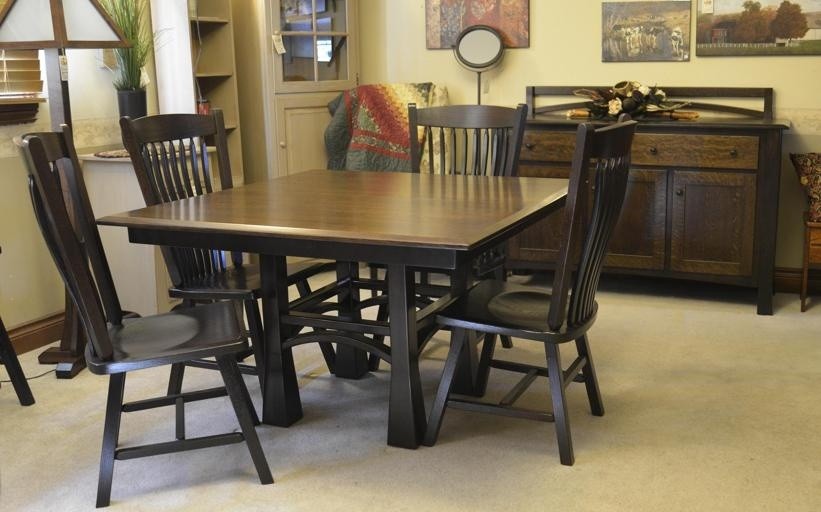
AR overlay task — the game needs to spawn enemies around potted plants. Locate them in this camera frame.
[93,1,163,150]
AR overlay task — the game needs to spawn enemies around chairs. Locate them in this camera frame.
[367,102,528,373]
[788,152,821,310]
[118,108,336,397]
[420,116,639,466]
[12,122,275,509]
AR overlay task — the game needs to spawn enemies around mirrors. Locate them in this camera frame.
[455,24,504,68]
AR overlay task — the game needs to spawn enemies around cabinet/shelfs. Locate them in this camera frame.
[74,144,218,317]
[272,92,345,263]
[149,0,245,195]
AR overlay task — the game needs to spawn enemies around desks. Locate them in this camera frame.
[95,168,571,450]
